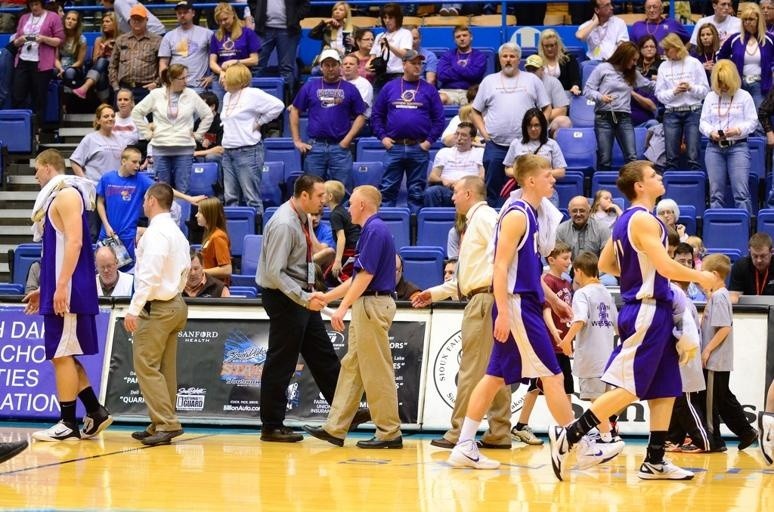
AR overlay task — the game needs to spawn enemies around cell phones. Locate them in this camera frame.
[717,130,724,136]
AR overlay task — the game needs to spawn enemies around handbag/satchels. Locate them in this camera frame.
[370,57,386,74]
[498,177,518,200]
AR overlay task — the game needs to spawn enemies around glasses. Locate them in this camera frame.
[174,75,191,83]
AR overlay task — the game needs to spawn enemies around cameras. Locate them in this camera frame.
[26,36,36,41]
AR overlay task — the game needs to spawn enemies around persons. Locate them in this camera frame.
[254,176,404,449]
[1,2,774,298]
[119,183,192,446]
[21,147,113,446]
[411,155,774,482]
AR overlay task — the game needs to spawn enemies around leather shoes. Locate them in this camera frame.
[260,407,403,449]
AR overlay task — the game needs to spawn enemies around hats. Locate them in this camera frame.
[401,49,424,62]
[172,1,193,10]
[524,55,543,69]
[128,5,147,18]
[318,49,342,63]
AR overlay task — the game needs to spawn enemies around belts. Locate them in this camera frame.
[390,137,425,146]
[665,106,700,112]
[464,286,494,299]
[119,81,149,90]
[708,137,747,149]
[360,290,393,296]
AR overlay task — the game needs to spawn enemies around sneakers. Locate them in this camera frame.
[71,88,86,100]
[1,405,183,463]
